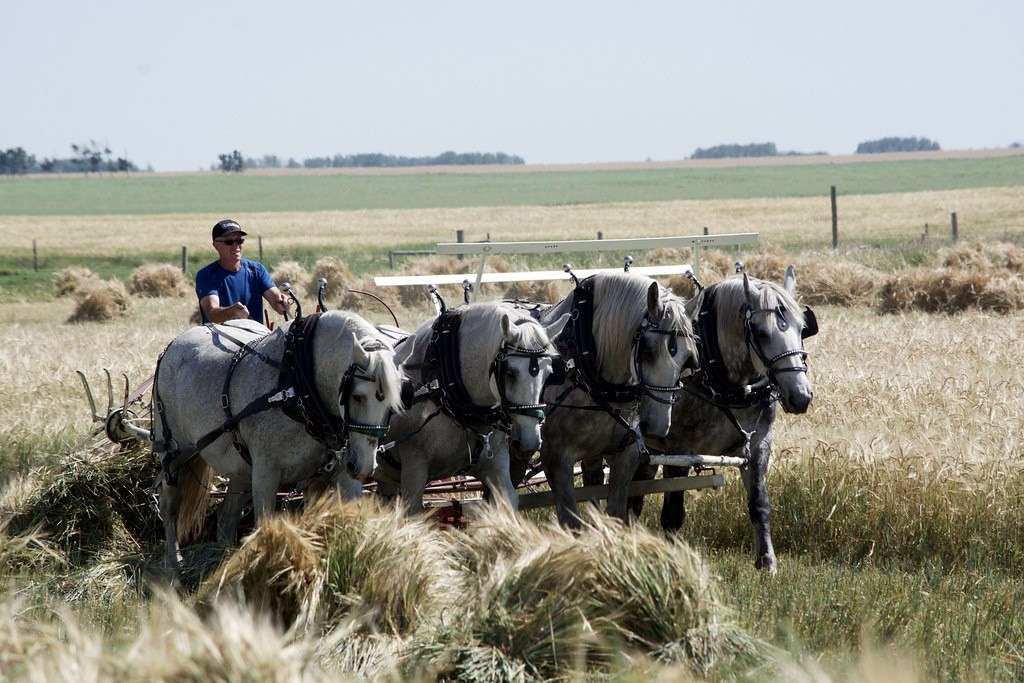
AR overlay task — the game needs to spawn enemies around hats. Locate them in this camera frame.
[212,219,247,242]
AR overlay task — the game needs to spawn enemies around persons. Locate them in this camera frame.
[195,220,292,324]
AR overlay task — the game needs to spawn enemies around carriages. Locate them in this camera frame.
[75,254,821,592]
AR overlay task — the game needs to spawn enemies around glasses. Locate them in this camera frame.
[214,239,244,246]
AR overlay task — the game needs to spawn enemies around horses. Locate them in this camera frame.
[147,264,814,578]
